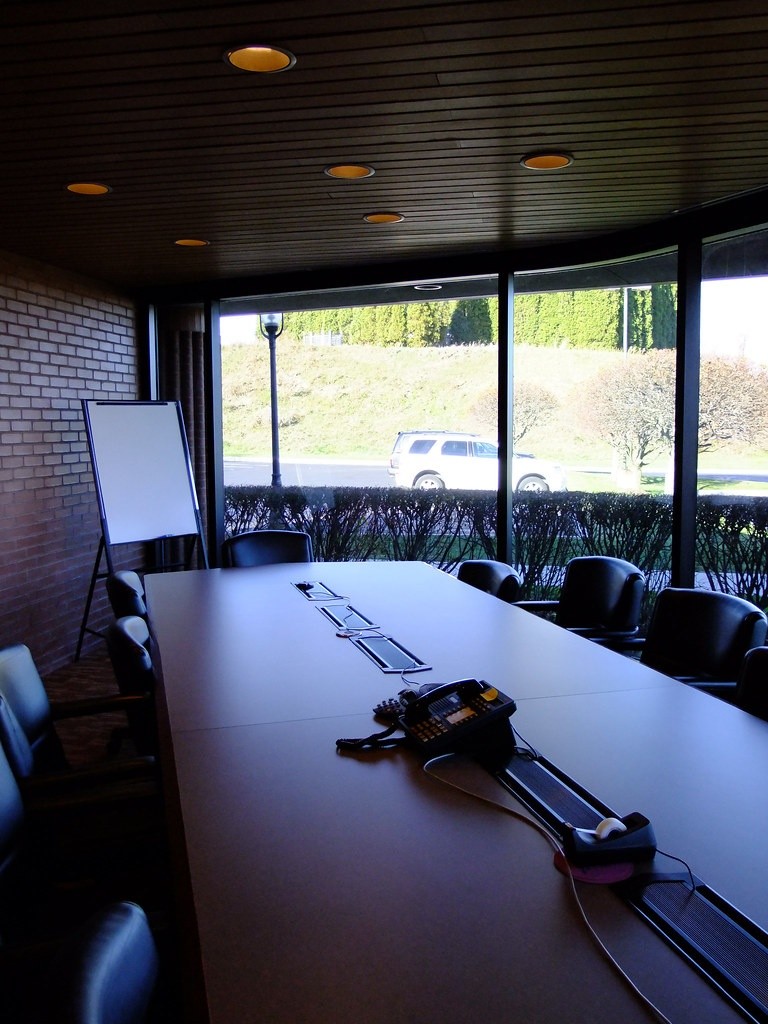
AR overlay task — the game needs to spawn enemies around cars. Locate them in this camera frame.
[387,429,567,494]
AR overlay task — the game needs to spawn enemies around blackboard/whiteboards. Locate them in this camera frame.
[80,398,203,546]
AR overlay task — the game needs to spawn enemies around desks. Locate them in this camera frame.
[142,559,767,1024]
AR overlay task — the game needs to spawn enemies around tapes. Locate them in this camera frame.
[574,817,628,842]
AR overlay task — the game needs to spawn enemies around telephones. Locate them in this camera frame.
[398,675,518,769]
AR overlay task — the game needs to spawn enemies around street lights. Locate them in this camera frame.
[261,313,291,530]
[605,285,652,351]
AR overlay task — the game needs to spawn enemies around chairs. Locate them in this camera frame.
[0,529,768,1024]
